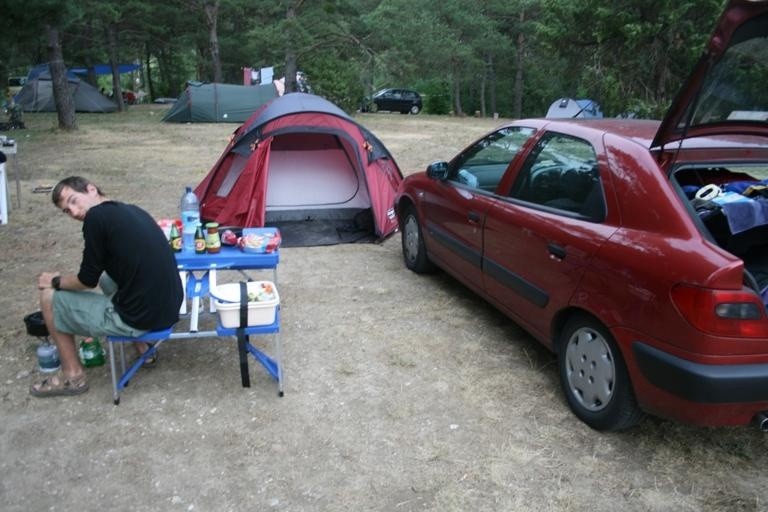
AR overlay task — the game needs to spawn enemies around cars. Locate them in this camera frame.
[109,91,135,105]
[361,88,422,115]
[393,0,768,431]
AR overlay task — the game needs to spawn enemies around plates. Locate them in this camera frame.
[209,283,259,303]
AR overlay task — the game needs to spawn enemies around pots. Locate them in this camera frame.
[24,311,48,337]
[218,227,242,237]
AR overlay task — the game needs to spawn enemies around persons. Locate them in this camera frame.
[29,177,184,398]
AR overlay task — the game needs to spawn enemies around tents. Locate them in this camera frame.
[194,92,404,248]
[546,97,602,119]
[17,68,120,114]
[161,79,279,122]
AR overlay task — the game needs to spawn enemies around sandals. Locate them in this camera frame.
[29,368,91,397]
[141,343,160,369]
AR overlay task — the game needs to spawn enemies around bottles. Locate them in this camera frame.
[180,186,201,251]
[205,222,221,254]
[170,224,182,253]
[194,223,206,254]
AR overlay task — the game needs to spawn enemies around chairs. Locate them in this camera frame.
[520,160,578,203]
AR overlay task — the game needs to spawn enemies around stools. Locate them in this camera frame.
[215,304,283,397]
[104,324,176,406]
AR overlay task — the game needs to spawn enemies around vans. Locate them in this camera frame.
[8,77,28,95]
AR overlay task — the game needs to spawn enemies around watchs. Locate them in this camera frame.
[51,276,61,291]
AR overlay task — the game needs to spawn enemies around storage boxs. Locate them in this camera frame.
[218,282,280,328]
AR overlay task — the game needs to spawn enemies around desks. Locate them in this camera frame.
[170,227,279,315]
